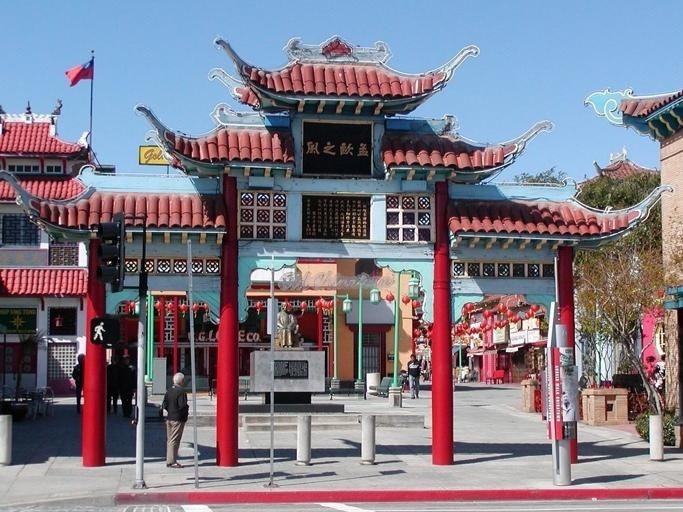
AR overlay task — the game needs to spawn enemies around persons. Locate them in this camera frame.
[406,353,421,400]
[421,355,471,386]
[118,352,136,418]
[161,371,190,468]
[275,301,297,349]
[70,353,85,416]
[106,355,121,415]
[395,369,407,395]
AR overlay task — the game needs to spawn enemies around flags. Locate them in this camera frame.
[64,59,91,88]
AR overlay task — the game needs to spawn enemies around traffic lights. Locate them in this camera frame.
[94,211,127,294]
[89,317,120,344]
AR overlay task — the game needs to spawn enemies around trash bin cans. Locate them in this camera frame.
[366,373,381,393]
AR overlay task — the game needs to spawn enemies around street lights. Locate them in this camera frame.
[457,336,464,384]
[353,278,381,401]
[386,268,420,406]
[331,292,354,396]
[133,294,152,381]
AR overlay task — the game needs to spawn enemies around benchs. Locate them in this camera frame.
[370,376,404,398]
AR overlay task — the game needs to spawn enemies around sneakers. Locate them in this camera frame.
[410,395,419,399]
[166,461,184,468]
